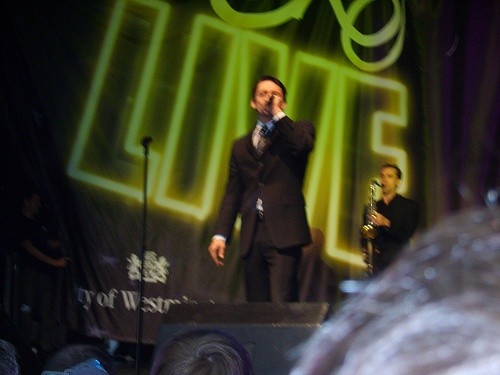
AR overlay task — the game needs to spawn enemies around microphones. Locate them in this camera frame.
[141,137,151,149]
[267,95,275,106]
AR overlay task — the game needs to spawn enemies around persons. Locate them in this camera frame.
[208,74,316,303]
[42,325,254,375]
[289,207,500,375]
[0,338,20,375]
[359,163,420,280]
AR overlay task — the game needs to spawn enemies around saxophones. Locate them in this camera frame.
[363,179,384,281]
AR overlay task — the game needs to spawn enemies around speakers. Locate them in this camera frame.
[154,302,334,375]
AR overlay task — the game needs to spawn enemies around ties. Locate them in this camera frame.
[256,125,269,154]
